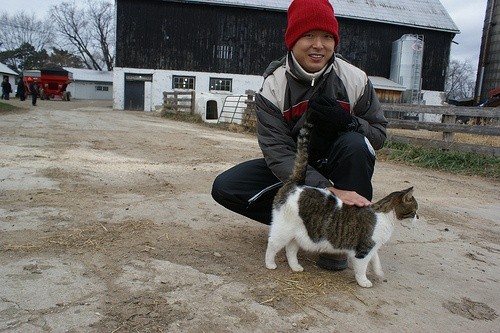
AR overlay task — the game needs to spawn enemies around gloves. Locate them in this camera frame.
[308,94,353,133]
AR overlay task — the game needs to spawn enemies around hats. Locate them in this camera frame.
[285,0,338,51]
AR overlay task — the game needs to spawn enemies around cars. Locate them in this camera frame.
[455,93,500,125]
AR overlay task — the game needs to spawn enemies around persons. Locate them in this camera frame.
[1,77,13,100]
[61,82,68,101]
[15,77,27,101]
[41,87,46,100]
[29,80,40,107]
[211,0,389,271]
[62,91,72,101]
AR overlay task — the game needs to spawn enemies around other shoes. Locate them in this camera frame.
[319,252,348,270]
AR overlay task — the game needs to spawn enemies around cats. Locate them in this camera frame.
[265,114,420,287]
